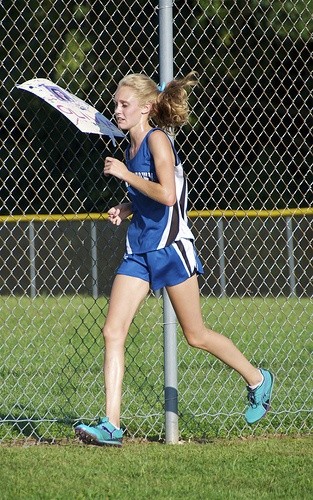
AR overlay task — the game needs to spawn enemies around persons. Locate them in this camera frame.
[74,71,273,447]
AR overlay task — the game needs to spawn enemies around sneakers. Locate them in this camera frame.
[75,419,123,447]
[245,368,274,424]
